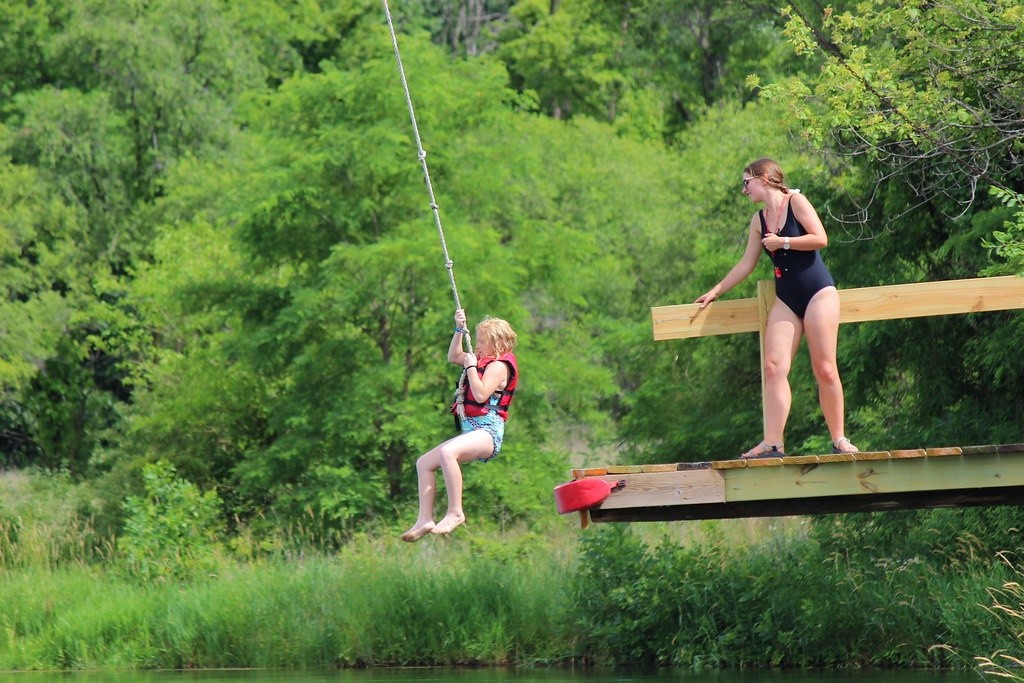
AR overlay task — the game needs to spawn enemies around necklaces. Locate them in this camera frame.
[767,194,786,233]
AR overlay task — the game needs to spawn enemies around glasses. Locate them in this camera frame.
[744,176,760,188]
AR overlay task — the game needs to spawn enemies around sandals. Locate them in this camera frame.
[832,437,858,453]
[738,441,785,459]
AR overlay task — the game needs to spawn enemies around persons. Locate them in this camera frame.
[400,308,518,542]
[694,158,859,460]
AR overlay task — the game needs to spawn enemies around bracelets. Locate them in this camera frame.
[466,365,477,370]
[455,327,462,332]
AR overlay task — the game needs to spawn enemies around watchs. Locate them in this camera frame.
[783,237,791,250]
